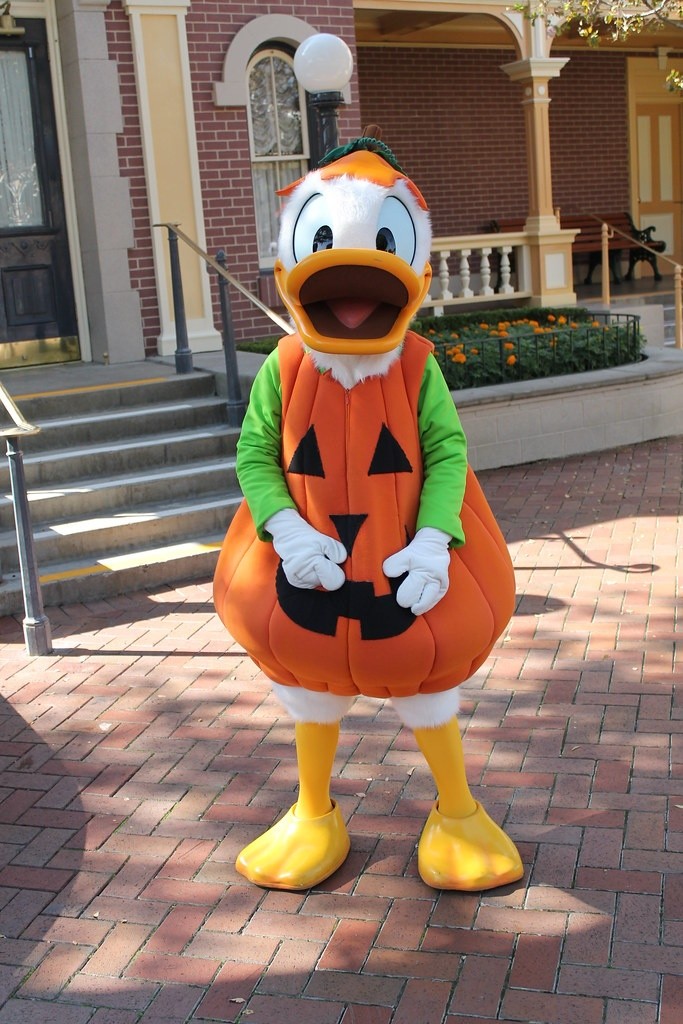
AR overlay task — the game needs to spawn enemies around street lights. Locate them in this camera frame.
[293,33,354,159]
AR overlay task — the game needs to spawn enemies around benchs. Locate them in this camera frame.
[490,209,666,283]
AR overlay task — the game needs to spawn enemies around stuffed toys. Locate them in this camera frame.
[210,135,525,892]
